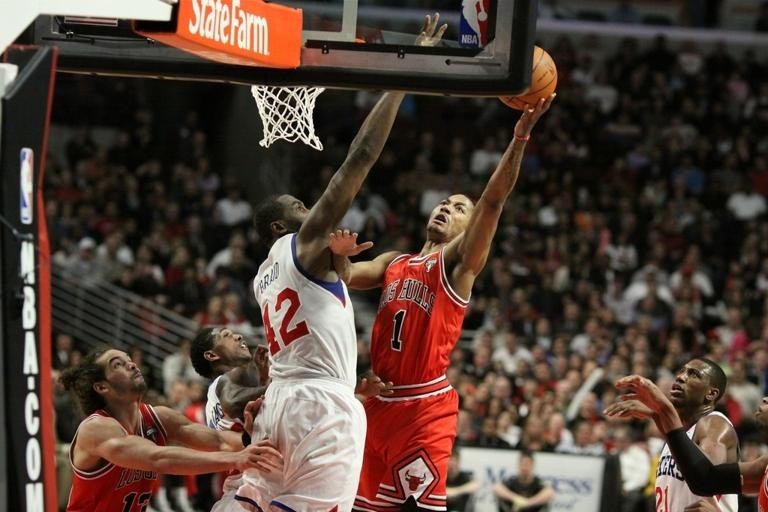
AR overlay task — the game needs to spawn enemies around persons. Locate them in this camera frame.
[36,0,768,512]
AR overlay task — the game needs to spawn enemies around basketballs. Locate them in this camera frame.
[498,46,557,110]
